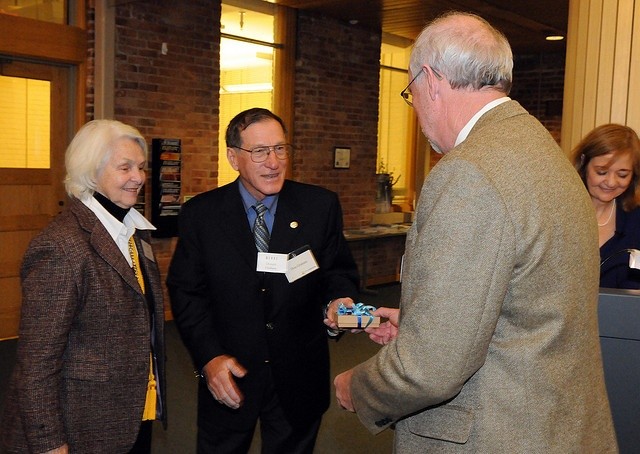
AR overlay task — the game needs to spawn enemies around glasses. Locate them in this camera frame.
[401,66,443,108]
[232,145,294,163]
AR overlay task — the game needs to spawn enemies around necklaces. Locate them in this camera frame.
[598,198,616,227]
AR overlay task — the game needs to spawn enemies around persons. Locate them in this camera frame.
[163,107,360,454]
[334,8,620,454]
[0,118,169,454]
[570,123,640,290]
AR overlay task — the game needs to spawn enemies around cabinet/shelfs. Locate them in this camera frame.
[152,137,182,239]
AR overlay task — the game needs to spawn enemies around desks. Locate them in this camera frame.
[339,222,413,297]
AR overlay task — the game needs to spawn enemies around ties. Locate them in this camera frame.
[251,202,271,252]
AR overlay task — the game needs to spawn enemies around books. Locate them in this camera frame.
[133,187,146,214]
[159,137,181,217]
